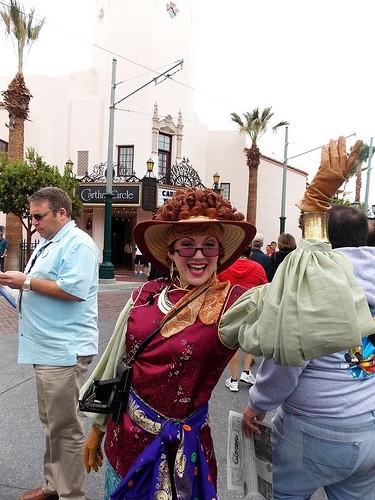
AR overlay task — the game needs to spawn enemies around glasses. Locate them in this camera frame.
[28,207,58,221]
[171,247,220,258]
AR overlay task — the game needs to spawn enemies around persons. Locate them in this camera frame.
[0,187,100,500]
[217,245,269,391]
[0,232,7,272]
[78,136,375,500]
[242,205,375,500]
[124,240,150,276]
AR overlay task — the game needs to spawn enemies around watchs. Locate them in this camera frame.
[22,276,33,292]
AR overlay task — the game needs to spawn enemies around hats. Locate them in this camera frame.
[132,187,256,275]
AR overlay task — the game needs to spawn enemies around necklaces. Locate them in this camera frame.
[157,285,175,315]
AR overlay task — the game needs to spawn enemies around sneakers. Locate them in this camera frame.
[239,369,257,385]
[225,375,239,391]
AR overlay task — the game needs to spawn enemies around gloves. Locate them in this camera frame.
[82,426,104,473]
[294,136,363,212]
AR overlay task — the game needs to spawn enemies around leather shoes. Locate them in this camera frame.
[20,486,59,500]
